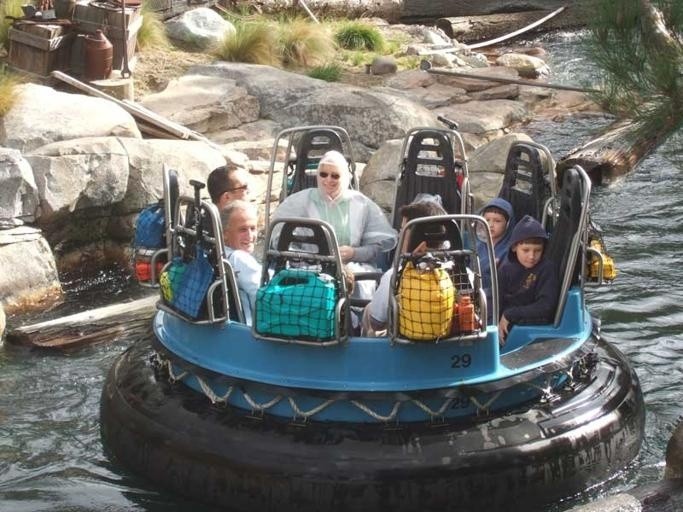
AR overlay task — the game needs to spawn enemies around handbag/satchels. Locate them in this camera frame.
[134,207,165,281]
[256,268,336,339]
[591,239,615,278]
[161,255,214,318]
[399,259,454,339]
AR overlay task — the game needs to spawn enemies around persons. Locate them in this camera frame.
[269,149,399,302]
[485,214,562,348]
[266,221,355,299]
[195,164,252,253]
[362,198,487,332]
[460,196,518,286]
[217,200,275,327]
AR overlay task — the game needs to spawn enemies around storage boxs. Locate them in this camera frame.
[4,0,143,87]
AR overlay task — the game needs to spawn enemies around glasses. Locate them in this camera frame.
[220,183,248,198]
[320,172,339,180]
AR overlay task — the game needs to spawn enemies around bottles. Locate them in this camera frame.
[167,256,188,296]
[458,296,474,332]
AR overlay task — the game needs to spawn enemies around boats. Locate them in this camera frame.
[91,123,645,512]
